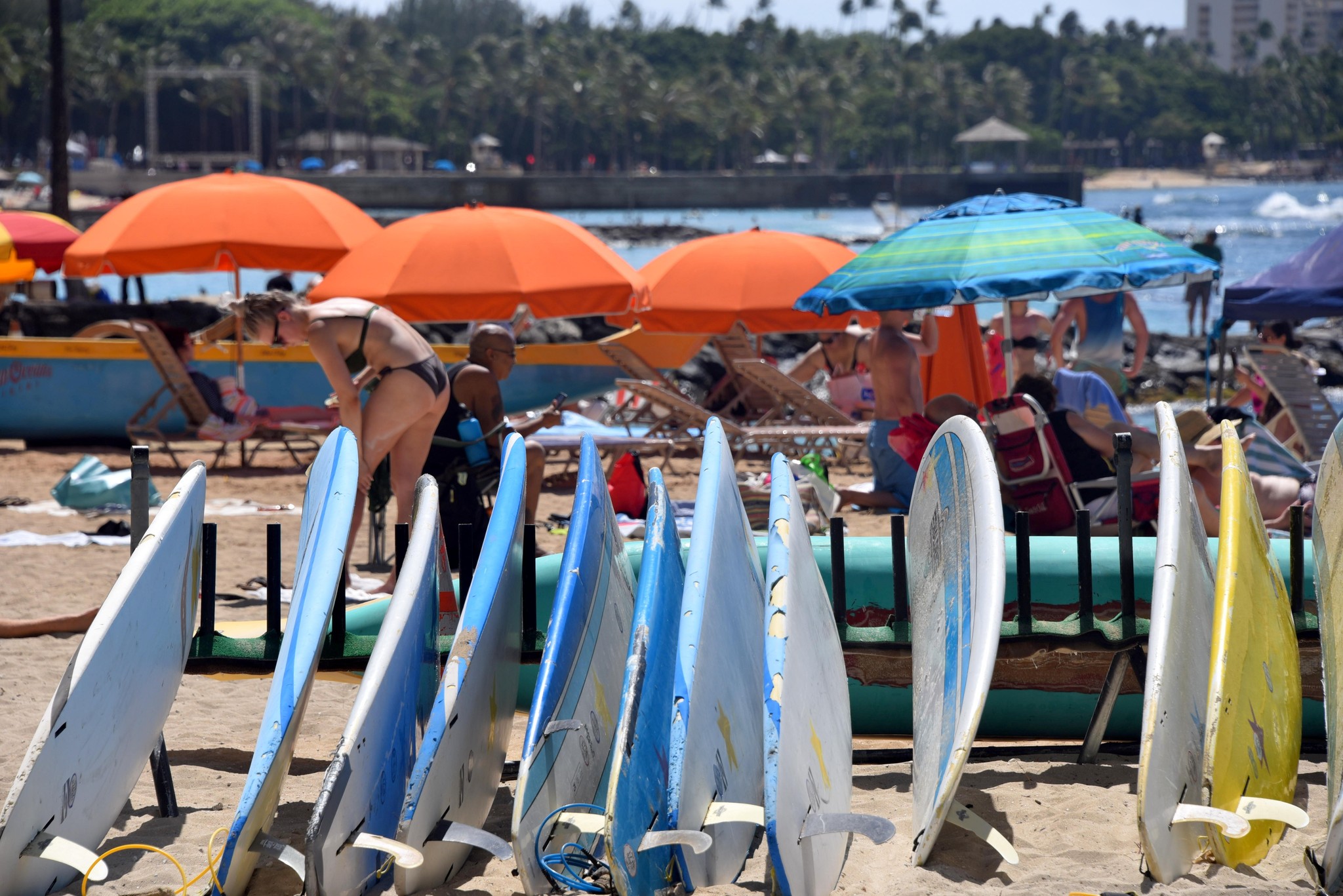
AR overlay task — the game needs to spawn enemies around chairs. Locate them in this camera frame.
[127,316,1343,541]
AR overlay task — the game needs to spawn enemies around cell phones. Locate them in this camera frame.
[1230,347,1238,367]
[554,392,567,411]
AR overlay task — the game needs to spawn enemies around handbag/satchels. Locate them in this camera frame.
[607,448,649,519]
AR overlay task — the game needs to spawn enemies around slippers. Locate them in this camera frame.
[236,576,293,590]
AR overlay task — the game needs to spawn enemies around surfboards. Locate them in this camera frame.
[1141,394,1247,882]
[211,424,359,896]
[669,416,762,892]
[1198,416,1309,871]
[905,412,1024,867]
[1307,418,1343,895]
[512,431,638,895]
[394,430,530,896]
[765,454,896,894]
[306,472,440,896]
[609,465,713,895]
[2,461,206,896]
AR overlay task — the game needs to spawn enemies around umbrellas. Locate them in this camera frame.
[0,206,82,284]
[1217,226,1343,321]
[60,169,384,393]
[792,194,1221,396]
[304,197,639,324]
[603,227,910,359]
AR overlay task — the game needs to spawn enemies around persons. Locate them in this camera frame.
[1181,227,1224,336]
[435,322,563,557]
[267,272,294,291]
[778,295,1339,537]
[115,188,149,305]
[223,288,452,593]
[162,326,354,429]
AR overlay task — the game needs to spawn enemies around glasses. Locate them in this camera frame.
[484,347,516,359]
[185,339,195,346]
[271,307,286,350]
[819,333,838,344]
[1258,332,1272,343]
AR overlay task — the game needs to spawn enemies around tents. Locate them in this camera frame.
[956,116,1029,176]
